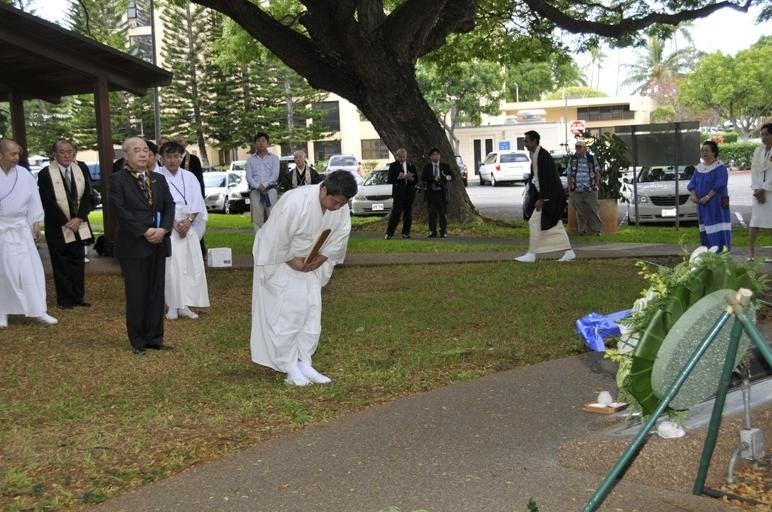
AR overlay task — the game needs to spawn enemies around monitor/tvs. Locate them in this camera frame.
[60,220,93,244]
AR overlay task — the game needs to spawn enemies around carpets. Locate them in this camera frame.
[66,170,72,193]
[139,175,147,197]
[435,165,437,188]
[400,164,405,185]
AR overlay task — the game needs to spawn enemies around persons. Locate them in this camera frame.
[117,135,208,257]
[284,152,321,191]
[0,140,60,329]
[154,142,210,318]
[251,167,359,386]
[385,151,419,239]
[516,124,772,266]
[38,142,98,308]
[107,136,178,353]
[421,150,451,239]
[246,134,280,234]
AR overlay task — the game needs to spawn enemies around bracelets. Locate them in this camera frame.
[187,217,193,223]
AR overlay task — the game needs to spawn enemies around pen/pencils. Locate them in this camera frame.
[230,160,250,176]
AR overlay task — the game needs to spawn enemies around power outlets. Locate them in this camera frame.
[549,150,571,174]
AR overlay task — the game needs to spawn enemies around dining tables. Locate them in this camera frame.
[559,131,635,233]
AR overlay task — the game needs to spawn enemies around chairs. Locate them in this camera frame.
[166,308,178,320]
[37,313,58,324]
[178,308,199,319]
[513,253,536,262]
[0,314,9,329]
[558,250,575,261]
[301,366,331,383]
[287,376,312,386]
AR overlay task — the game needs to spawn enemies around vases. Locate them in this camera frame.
[606,247,769,440]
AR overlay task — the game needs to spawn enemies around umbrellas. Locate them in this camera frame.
[160,343,174,350]
[427,231,437,238]
[747,257,755,261]
[764,257,772,262]
[440,233,447,238]
[63,304,73,308]
[402,234,410,238]
[385,234,391,239]
[133,346,146,354]
[73,302,90,306]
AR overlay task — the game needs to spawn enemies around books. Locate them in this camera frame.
[60,220,93,244]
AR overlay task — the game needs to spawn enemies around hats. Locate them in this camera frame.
[477,150,530,185]
[351,169,393,216]
[202,172,248,213]
[628,160,703,223]
[454,155,468,185]
[228,177,251,214]
[326,154,362,177]
[89,187,101,209]
[281,157,315,173]
[693,117,747,132]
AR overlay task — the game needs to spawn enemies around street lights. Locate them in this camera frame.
[721,196,729,208]
[208,248,233,267]
